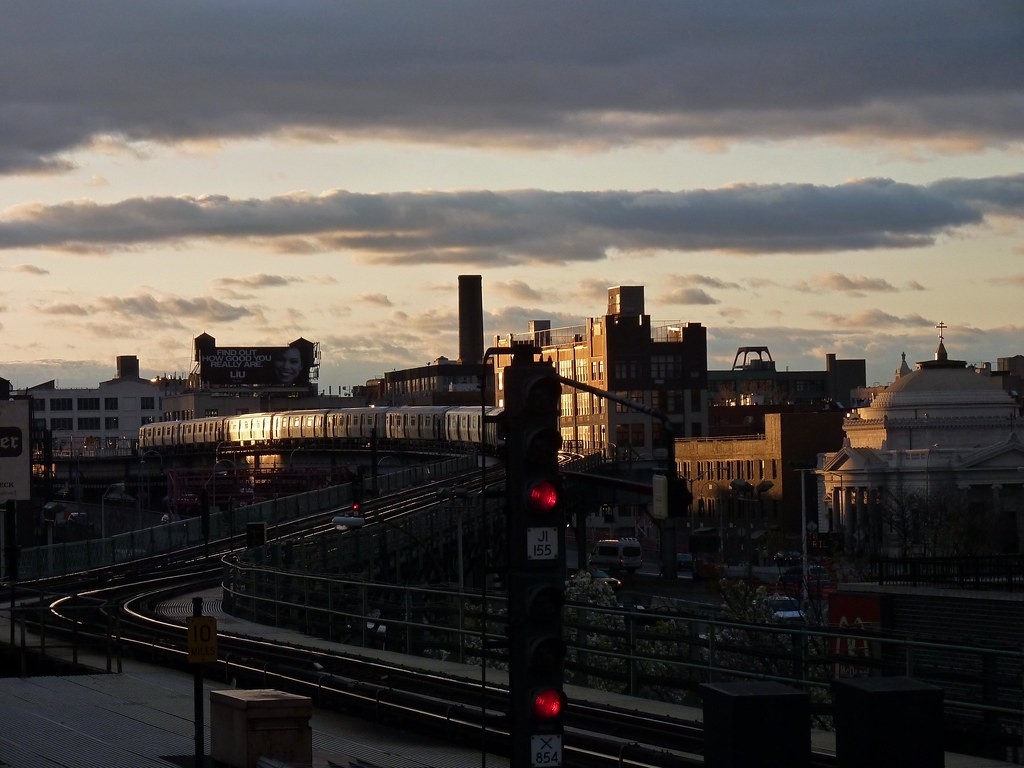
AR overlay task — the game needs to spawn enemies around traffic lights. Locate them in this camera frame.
[516,590,568,734]
[352,504,361,511]
[508,363,563,517]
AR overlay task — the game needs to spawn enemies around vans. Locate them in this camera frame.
[589,538,642,574]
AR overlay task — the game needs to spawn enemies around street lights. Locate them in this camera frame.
[102,483,125,563]
[331,517,442,586]
[731,479,774,581]
[926,444,941,501]
[678,467,729,536]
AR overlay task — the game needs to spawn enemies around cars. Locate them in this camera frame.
[763,596,806,623]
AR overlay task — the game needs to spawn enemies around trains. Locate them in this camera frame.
[138,405,507,455]
